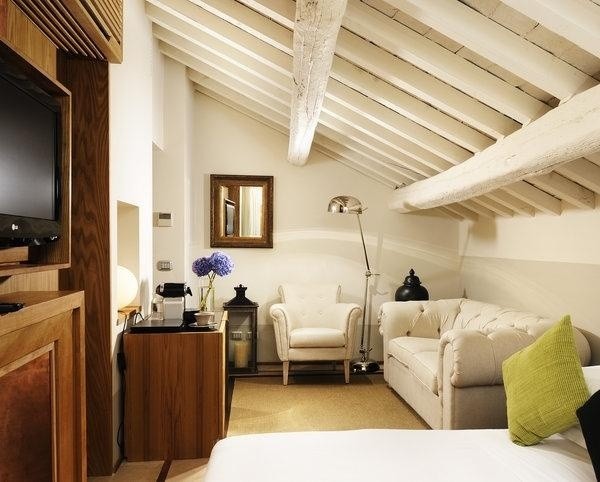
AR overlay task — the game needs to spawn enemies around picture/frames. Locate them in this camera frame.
[210,174,274,248]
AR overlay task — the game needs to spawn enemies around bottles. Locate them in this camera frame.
[150,292,164,321]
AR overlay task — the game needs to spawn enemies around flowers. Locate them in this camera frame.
[192,251,235,311]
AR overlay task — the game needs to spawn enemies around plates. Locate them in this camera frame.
[188,321,218,328]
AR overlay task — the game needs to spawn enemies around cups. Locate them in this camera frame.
[194,312,211,326]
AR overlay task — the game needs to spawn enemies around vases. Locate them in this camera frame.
[200,285,216,322]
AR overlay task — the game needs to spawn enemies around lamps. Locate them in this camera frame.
[328,195,380,375]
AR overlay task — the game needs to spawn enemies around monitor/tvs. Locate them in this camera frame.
[225,203,234,235]
[0,57,62,248]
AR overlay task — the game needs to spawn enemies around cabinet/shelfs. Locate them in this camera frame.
[0,290,88,482]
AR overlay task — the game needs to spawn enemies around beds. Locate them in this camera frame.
[200,365,599,482]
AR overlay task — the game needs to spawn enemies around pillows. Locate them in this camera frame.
[503,314,600,477]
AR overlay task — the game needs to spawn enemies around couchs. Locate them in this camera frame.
[269,279,363,386]
[377,297,592,430]
